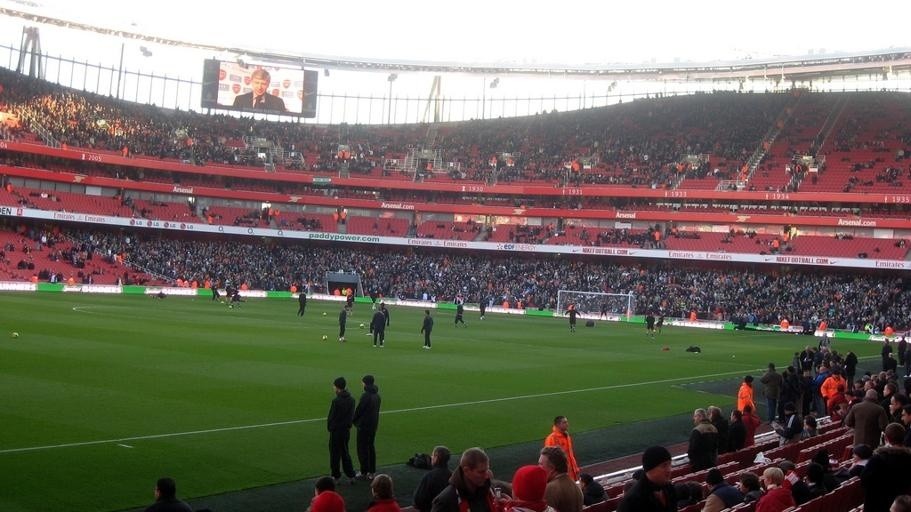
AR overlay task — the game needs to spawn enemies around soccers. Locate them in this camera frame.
[12,332,19,336]
[323,313,327,315]
[323,335,327,339]
[360,323,364,327]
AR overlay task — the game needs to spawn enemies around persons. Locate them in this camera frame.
[144,478,193,512]
[309,476,347,512]
[326,378,358,485]
[366,474,401,512]
[618,334,910,511]
[353,376,380,480]
[1,64,911,348]
[414,416,609,511]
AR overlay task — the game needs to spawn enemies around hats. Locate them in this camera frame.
[853,443,873,459]
[512,465,547,501]
[643,446,671,473]
[312,491,345,512]
[706,469,723,484]
[334,377,346,389]
[362,375,374,385]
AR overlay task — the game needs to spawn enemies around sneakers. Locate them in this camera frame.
[339,333,432,350]
[331,472,376,485]
